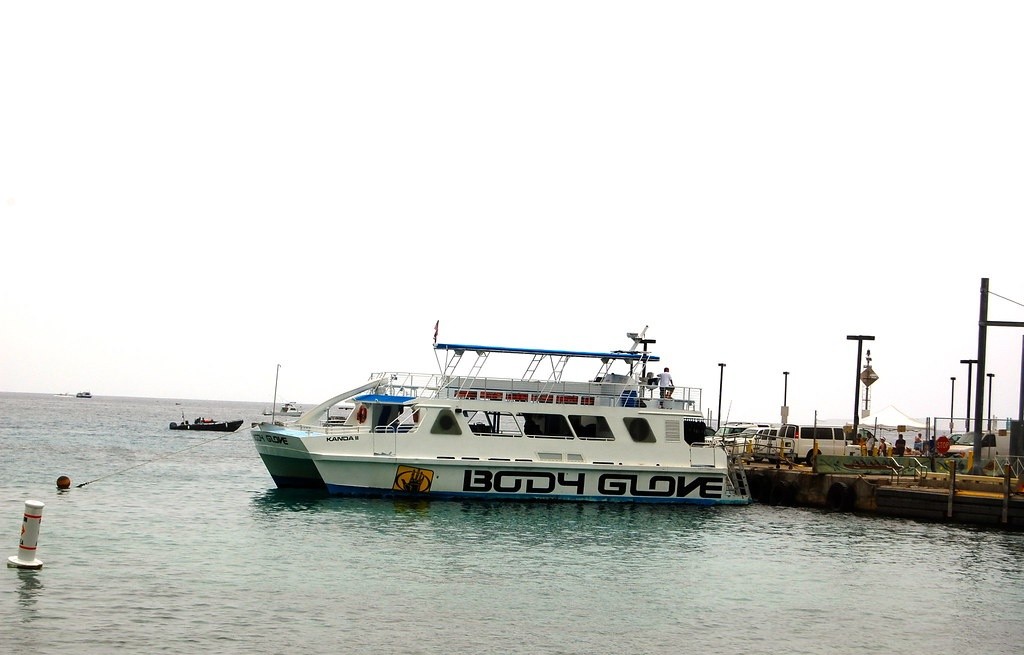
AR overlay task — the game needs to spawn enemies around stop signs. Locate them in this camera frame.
[934,436,951,454]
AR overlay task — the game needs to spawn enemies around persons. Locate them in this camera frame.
[657,367,675,408]
[194,417,214,423]
[857,431,936,456]
[522,414,542,434]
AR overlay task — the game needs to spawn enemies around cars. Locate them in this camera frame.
[947,430,1019,472]
[777,426,860,462]
[712,422,777,461]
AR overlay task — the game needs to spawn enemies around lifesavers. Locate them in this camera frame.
[359,405,367,423]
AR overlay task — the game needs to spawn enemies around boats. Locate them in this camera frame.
[278,404,301,417]
[171,417,243,433]
[76,392,92,398]
[251,338,753,509]
